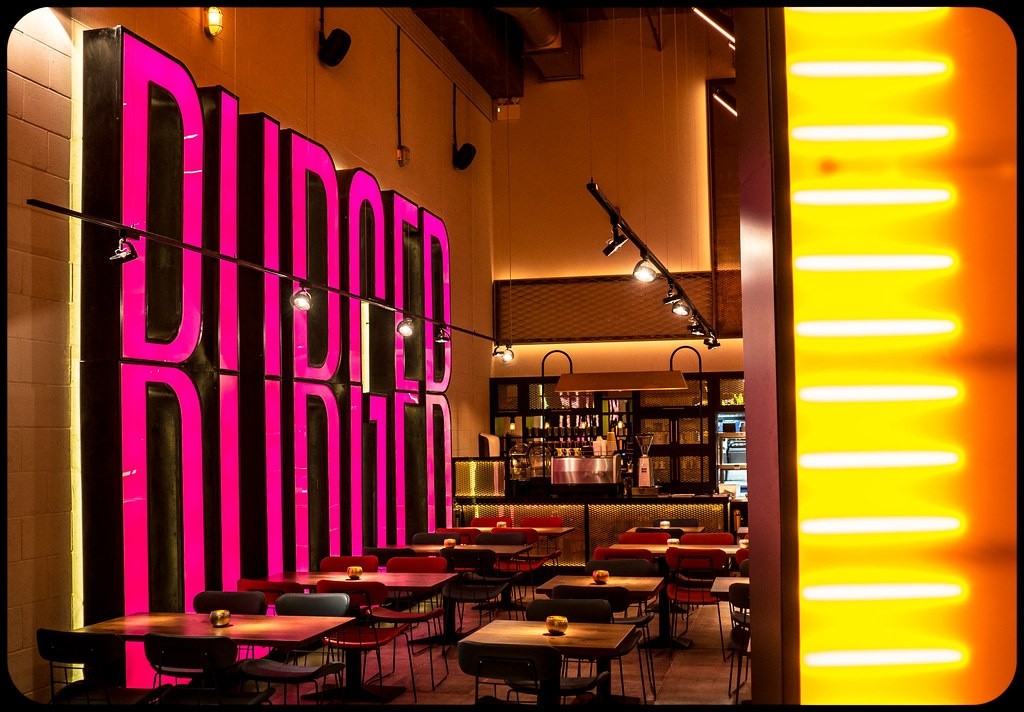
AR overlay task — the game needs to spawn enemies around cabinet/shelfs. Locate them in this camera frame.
[715,412,748,502]
[490,377,638,488]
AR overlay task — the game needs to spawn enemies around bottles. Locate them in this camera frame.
[529,438,551,477]
[509,438,530,481]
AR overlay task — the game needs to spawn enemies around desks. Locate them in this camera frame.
[260,571,461,706]
[456,619,636,706]
[711,576,751,596]
[68,610,355,642]
[447,527,575,611]
[388,545,533,646]
[534,575,665,706]
[624,526,707,532]
[608,544,740,653]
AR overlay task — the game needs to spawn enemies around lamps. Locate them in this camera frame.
[492,349,514,362]
[109,236,138,266]
[397,317,417,337]
[662,294,691,316]
[290,288,314,312]
[602,233,629,258]
[687,325,722,350]
[435,329,452,345]
[633,259,657,283]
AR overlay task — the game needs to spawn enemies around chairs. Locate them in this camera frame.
[35,516,751,712]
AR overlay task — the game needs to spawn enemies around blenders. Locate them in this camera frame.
[635,434,654,487]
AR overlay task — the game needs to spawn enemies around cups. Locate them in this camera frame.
[444,539,456,549]
[607,432,615,455]
[209,609,231,627]
[347,565,363,580]
[545,615,568,635]
[667,538,679,548]
[497,521,506,528]
[739,539,749,548]
[660,521,671,529]
[592,570,609,585]
